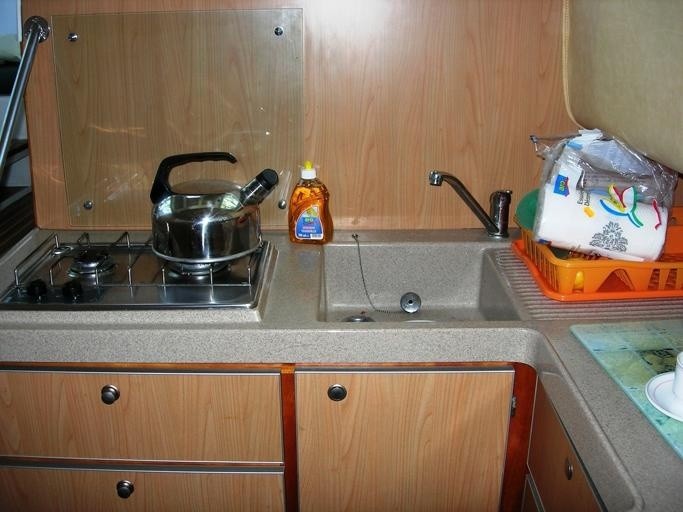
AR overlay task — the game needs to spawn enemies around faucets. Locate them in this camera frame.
[427,168,513,239]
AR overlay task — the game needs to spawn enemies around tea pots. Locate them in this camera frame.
[148,151,278,261]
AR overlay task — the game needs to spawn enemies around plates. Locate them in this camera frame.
[645,372,683,423]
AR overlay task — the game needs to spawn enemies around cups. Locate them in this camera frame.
[670,351,683,399]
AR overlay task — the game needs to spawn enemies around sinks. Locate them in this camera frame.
[313,240,527,326]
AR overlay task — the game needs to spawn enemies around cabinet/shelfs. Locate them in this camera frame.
[0,357,290,512]
[283,359,525,512]
[516,364,614,511]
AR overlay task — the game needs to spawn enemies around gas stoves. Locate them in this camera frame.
[0,232,278,310]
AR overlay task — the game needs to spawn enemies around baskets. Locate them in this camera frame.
[512,206,683,291]
